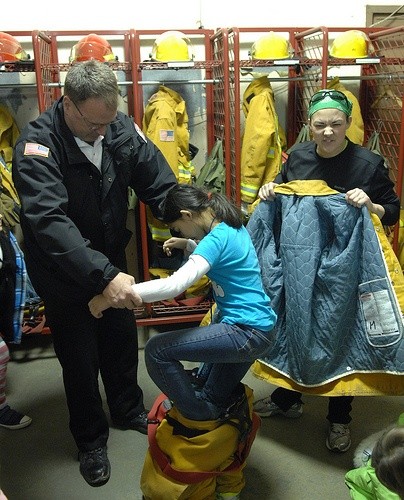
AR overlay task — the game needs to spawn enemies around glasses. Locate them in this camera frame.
[69,95,121,130]
[309,90,349,109]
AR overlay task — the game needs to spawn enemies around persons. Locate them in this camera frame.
[246,90,404,453]
[0,335,33,429]
[371,427,404,497]
[12,59,179,486]
[87,183,278,500]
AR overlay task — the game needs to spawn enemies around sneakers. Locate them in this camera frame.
[253,396,302,418]
[0,406,32,429]
[326,421,351,452]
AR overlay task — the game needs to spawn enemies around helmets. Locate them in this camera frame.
[73,33,117,63]
[329,30,368,59]
[252,34,289,59]
[154,36,189,61]
[0,32,30,62]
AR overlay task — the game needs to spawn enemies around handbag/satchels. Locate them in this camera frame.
[196,141,225,196]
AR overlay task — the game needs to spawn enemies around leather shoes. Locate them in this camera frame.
[115,409,152,434]
[78,445,111,486]
